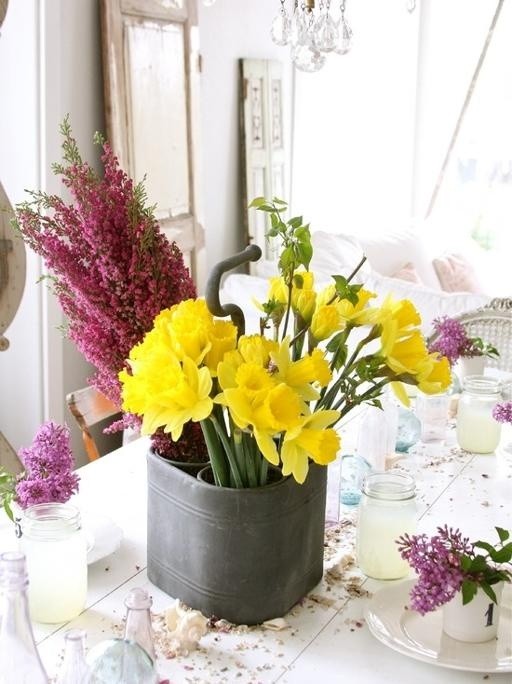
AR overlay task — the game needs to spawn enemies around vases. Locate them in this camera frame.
[452,355,484,380]
[147,446,327,626]
[442,580,504,642]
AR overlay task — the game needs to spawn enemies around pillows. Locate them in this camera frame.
[393,261,422,285]
[433,246,487,291]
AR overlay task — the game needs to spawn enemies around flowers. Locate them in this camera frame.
[118,197,453,489]
[428,314,500,366]
[395,524,512,617]
[0,423,81,523]
[10,114,209,462]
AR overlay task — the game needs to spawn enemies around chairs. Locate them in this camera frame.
[427,298,512,371]
[66,385,121,461]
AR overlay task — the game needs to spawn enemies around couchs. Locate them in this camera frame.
[309,229,512,343]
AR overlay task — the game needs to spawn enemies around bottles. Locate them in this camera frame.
[415,390,448,442]
[121,588,157,684]
[53,632,88,684]
[0,551,50,684]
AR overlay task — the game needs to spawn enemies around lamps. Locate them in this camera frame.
[270,0,352,72]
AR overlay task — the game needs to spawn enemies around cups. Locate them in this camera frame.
[325,458,342,530]
[417,388,452,443]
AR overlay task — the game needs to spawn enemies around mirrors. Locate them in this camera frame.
[100,0,204,255]
